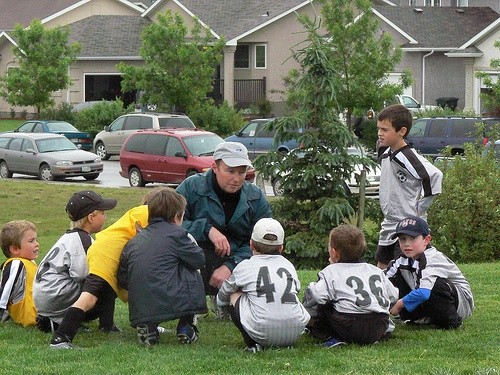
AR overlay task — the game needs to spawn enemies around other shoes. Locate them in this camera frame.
[249,344,262,353]
[77,323,90,332]
[99,326,122,333]
[322,339,342,347]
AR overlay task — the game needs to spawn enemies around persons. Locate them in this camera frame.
[383,217,475,329]
[51,185,173,349]
[0,220,41,327]
[303,224,400,348]
[32,190,123,334]
[117,189,206,345]
[374,104,444,270]
[217,217,311,354]
[175,141,275,320]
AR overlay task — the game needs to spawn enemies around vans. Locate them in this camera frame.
[93,111,198,161]
[405,116,500,162]
[118,127,255,188]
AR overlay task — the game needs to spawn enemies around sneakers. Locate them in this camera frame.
[193,313,200,326]
[50,334,75,349]
[139,329,161,345]
[215,297,232,322]
[176,323,199,345]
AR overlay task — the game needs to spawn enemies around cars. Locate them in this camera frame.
[223,118,308,161]
[271,147,381,199]
[366,95,443,119]
[0,119,104,181]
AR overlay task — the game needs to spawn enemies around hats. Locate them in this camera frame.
[251,218,284,245]
[65,190,117,221]
[391,217,430,240]
[213,141,254,170]
